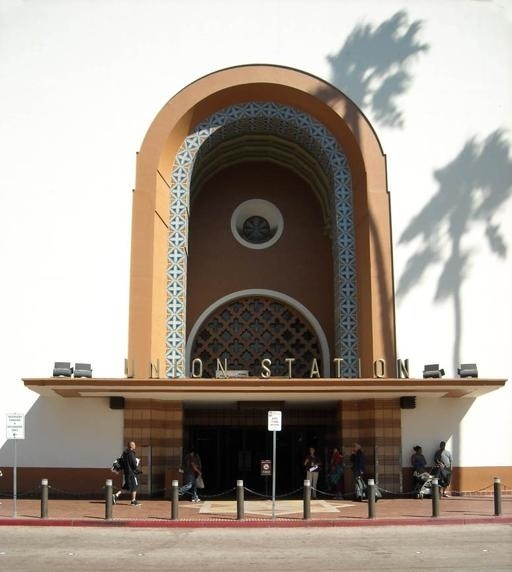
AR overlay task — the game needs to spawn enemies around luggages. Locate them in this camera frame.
[415,461,437,499]
[365,486,382,503]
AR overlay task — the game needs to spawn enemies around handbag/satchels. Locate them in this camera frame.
[336,464,345,475]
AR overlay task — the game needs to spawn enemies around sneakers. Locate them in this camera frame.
[112,494,117,505]
[196,475,204,489]
[131,500,142,506]
[443,492,453,497]
[192,498,200,503]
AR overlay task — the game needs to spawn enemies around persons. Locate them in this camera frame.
[303,445,322,500]
[329,445,346,499]
[179,448,203,502]
[112,441,142,507]
[433,440,455,498]
[410,444,427,499]
[350,439,369,501]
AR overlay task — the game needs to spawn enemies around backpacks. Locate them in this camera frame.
[352,473,368,499]
[111,457,124,475]
